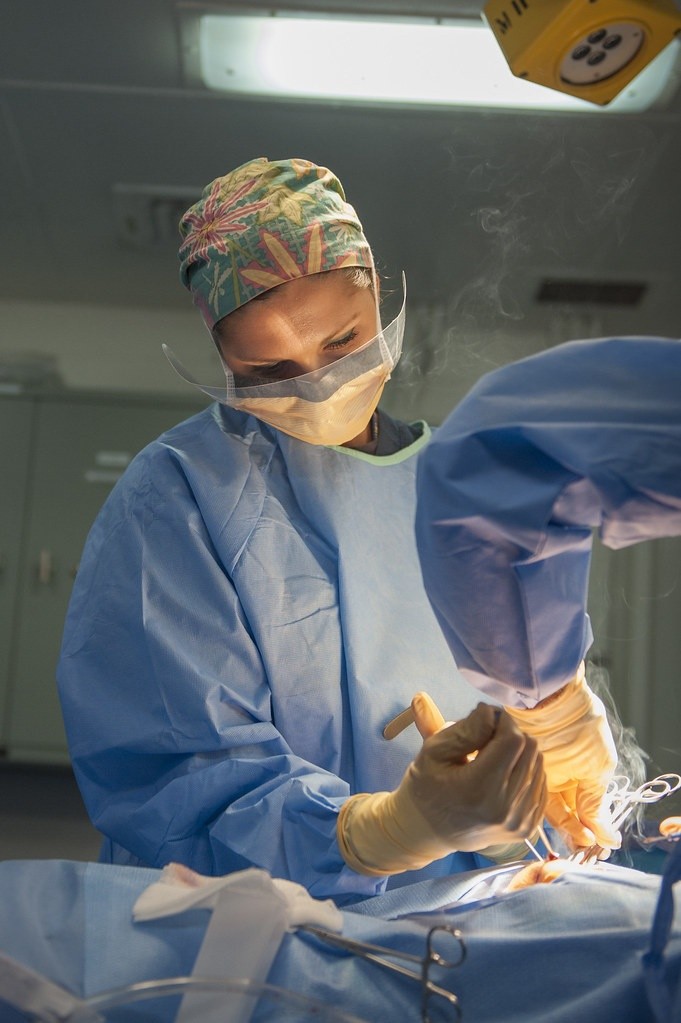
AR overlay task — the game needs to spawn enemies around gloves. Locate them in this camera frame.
[504,659,623,852]
[337,702,549,879]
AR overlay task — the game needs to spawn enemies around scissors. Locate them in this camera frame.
[298,923,468,1023]
[567,773,681,865]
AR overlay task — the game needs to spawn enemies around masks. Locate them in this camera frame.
[210,268,394,446]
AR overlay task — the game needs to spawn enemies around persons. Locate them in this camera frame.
[415,337,681,864]
[59,158,568,905]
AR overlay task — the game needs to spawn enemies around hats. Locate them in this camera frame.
[177,156,375,329]
